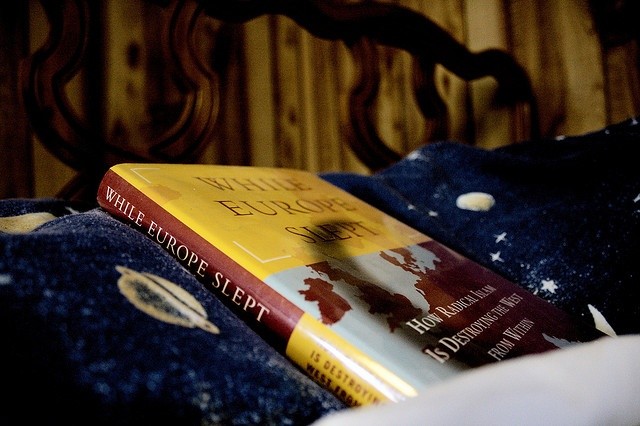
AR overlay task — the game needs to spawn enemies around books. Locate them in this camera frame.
[97,164,609,405]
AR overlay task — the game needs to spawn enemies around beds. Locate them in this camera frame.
[0,0,640,426]
